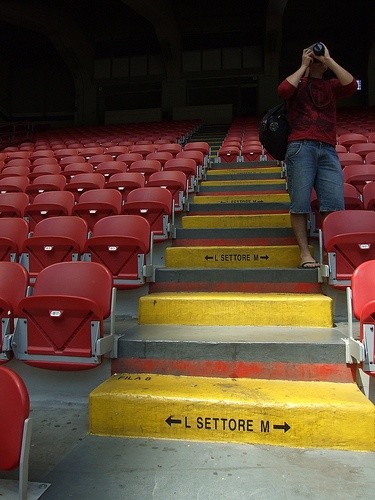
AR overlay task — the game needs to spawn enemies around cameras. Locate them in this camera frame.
[308,43,324,63]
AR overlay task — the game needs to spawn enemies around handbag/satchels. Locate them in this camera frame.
[259,102,289,161]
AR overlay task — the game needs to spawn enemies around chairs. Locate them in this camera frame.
[0,104,375,500]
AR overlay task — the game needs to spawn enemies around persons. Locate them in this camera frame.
[278,42,358,268]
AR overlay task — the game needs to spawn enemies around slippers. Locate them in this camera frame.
[298,261,319,269]
